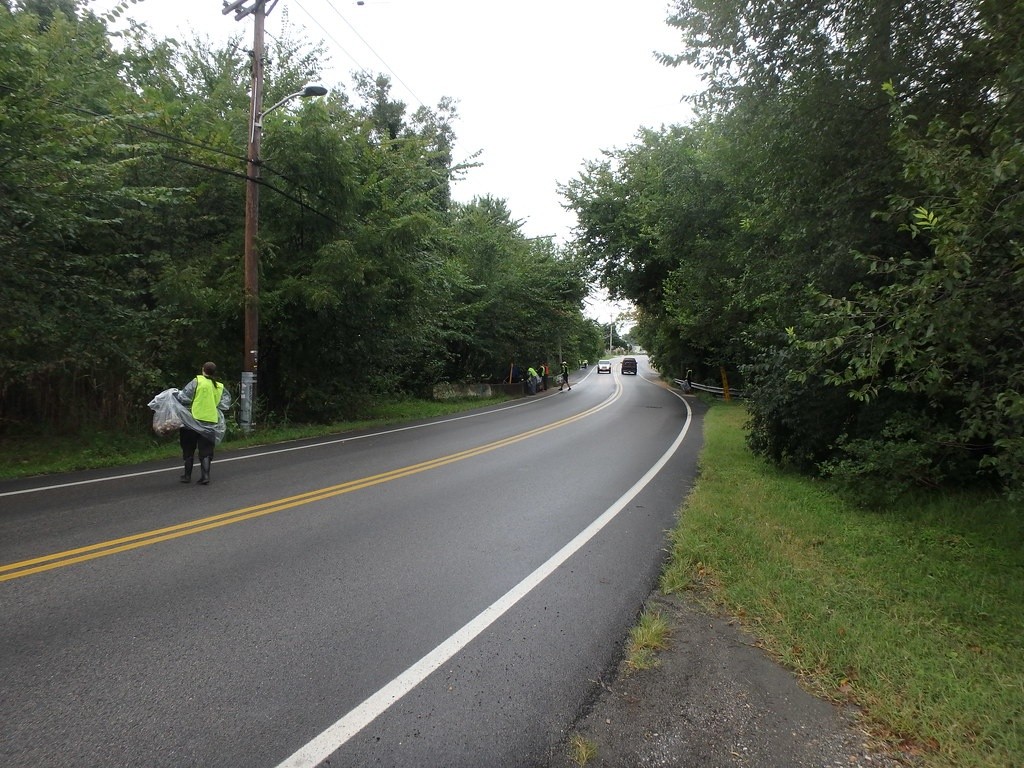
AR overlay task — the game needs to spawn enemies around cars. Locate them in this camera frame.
[597,360,612,374]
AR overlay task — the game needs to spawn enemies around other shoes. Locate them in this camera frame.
[558,388,563,390]
[568,388,571,391]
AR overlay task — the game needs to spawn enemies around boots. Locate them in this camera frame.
[180,457,193,483]
[196,456,210,484]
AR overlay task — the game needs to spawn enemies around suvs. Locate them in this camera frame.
[621,358,638,375]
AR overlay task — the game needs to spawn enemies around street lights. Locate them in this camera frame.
[239,86,329,437]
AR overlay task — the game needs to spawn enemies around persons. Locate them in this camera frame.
[173,361,230,484]
[521,359,588,395]
[685,366,692,387]
